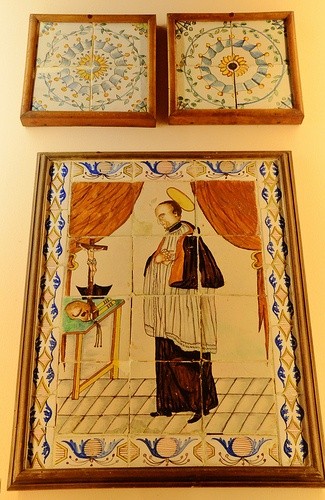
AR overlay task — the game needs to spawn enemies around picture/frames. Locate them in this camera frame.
[166,11,306,126]
[6,149,323,490]
[20,12,158,128]
